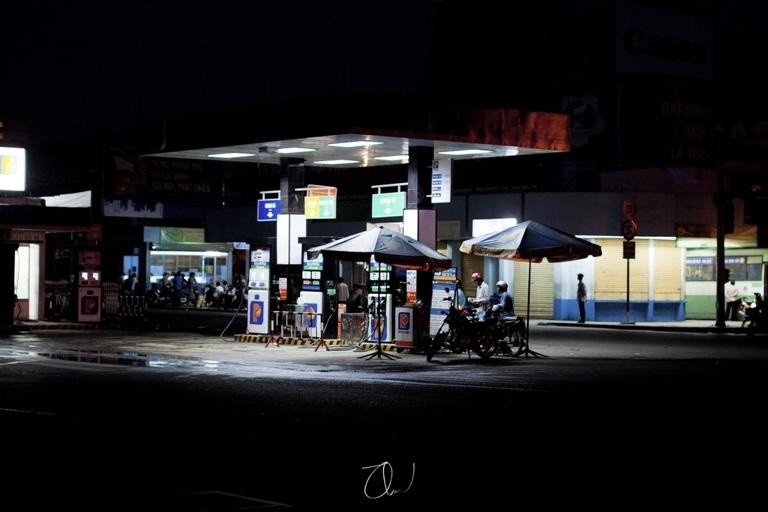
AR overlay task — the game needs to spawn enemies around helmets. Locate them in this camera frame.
[495,281,508,290]
[470,272,483,283]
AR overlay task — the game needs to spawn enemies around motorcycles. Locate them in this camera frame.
[205,285,246,311]
[467,296,527,359]
[737,292,767,337]
[149,284,189,308]
[425,286,502,362]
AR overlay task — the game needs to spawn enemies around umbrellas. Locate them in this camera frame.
[458,218,602,357]
[306,226,453,360]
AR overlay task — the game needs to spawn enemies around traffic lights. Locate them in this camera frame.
[722,201,735,234]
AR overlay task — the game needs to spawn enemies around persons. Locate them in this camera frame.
[336,277,349,304]
[467,272,490,321]
[577,274,586,323]
[730,280,741,321]
[752,292,764,317]
[493,281,514,318]
[122,266,248,309]
[454,278,466,309]
[725,278,737,320]
